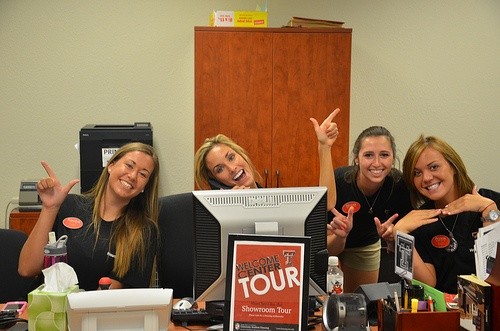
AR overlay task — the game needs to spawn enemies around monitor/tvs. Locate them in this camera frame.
[191,186,329,331]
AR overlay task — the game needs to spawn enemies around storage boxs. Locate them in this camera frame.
[79,122,153,195]
[214,10,268,28]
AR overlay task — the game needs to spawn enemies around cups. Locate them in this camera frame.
[404,284,424,309]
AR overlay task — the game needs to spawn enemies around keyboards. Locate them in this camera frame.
[170,308,219,326]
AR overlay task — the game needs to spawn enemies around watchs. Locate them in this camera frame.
[480,209,500,222]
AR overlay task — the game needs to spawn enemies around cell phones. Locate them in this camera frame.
[1,301,27,315]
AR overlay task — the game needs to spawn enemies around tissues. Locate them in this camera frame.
[27,262,85,331]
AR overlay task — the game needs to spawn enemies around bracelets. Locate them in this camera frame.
[481,201,496,213]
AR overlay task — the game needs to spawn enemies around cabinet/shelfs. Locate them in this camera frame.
[193,26,353,191]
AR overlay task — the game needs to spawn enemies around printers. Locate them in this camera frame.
[79,122,154,196]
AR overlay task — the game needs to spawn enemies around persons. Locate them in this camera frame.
[393,136,500,294]
[195,107,341,212]
[18,142,161,291]
[326,125,412,293]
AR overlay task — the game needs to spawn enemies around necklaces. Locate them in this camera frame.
[359,180,384,213]
[439,213,457,253]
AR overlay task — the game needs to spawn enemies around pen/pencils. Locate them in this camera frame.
[386,289,400,313]
[427,295,436,311]
[404,290,408,310]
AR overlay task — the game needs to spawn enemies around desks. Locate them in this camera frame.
[0,292,379,331]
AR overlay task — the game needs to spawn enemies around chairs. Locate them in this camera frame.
[0,228,44,305]
[151,191,196,299]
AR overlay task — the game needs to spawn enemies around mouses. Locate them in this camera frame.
[173,297,198,310]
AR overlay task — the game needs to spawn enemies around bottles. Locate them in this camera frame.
[326,256,344,297]
[42,232,68,268]
